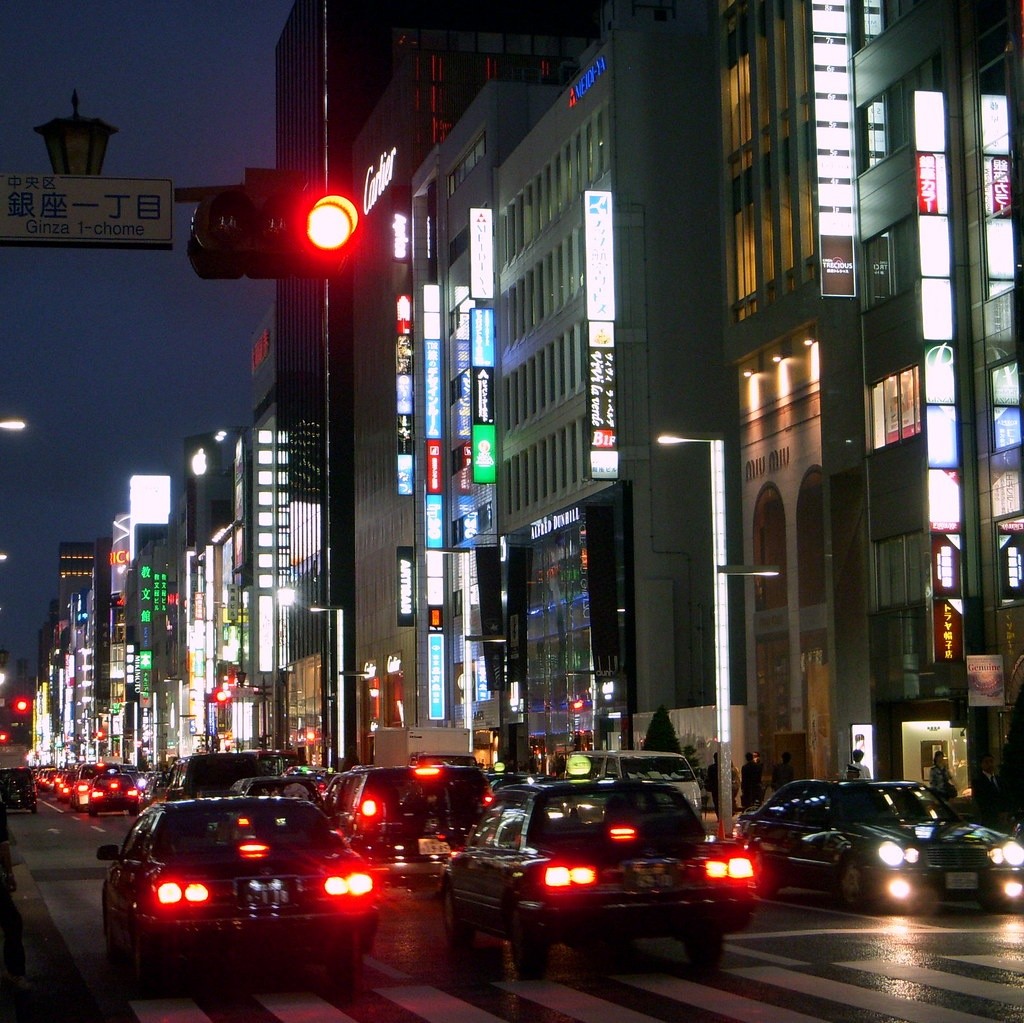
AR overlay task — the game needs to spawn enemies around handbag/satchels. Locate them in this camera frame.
[943,781,958,799]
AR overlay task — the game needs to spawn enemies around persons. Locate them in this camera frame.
[602,796,662,857]
[929,751,953,802]
[844,750,871,779]
[973,754,1011,825]
[705,752,767,822]
[771,752,794,791]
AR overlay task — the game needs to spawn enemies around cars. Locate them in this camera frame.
[96,797,379,979]
[283,765,328,788]
[490,772,546,786]
[437,779,760,977]
[735,779,1024,910]
[35,762,168,813]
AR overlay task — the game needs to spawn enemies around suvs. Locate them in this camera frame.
[216,778,327,844]
[156,753,269,802]
[322,767,498,890]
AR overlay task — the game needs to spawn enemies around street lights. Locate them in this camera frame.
[237,669,268,752]
[312,607,371,772]
[659,434,779,844]
[427,546,508,753]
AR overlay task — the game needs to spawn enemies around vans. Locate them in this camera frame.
[562,750,702,815]
[374,725,484,769]
[0,766,38,812]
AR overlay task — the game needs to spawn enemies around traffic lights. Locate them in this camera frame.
[191,189,389,279]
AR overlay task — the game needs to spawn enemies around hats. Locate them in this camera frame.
[847,762,861,773]
